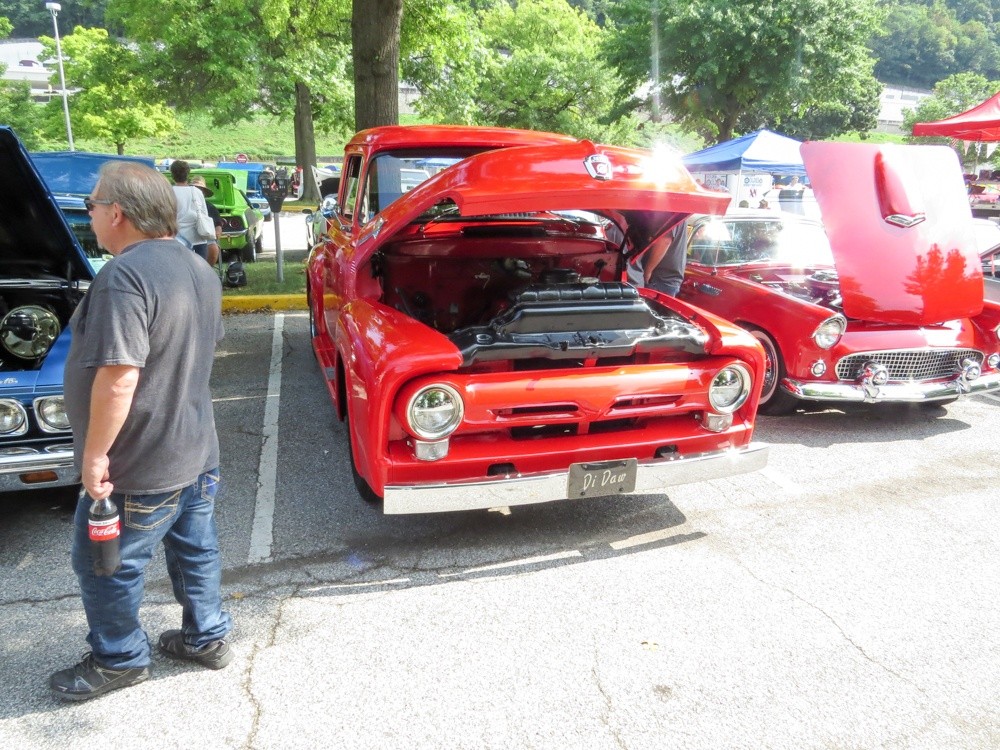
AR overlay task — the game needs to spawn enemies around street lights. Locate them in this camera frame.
[46,2,76,152]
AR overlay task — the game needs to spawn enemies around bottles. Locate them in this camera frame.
[88,482,122,579]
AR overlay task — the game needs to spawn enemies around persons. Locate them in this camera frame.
[46,159,232,701]
[714,178,728,191]
[785,176,805,191]
[641,220,687,298]
[170,161,222,267]
[696,179,709,190]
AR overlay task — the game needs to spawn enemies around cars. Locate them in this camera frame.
[0,124,156,502]
[160,168,265,289]
[673,140,1000,418]
[972,215,999,286]
[306,123,768,516]
[159,158,451,222]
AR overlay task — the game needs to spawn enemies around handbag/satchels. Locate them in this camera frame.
[226,262,246,286]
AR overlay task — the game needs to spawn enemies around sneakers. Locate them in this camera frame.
[49,652,149,701]
[159,629,234,670]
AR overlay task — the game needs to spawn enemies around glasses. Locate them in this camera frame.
[84,198,112,210]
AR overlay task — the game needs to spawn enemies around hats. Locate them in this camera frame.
[188,175,213,197]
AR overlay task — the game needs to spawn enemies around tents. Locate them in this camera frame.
[683,128,819,213]
[912,91,1000,180]
